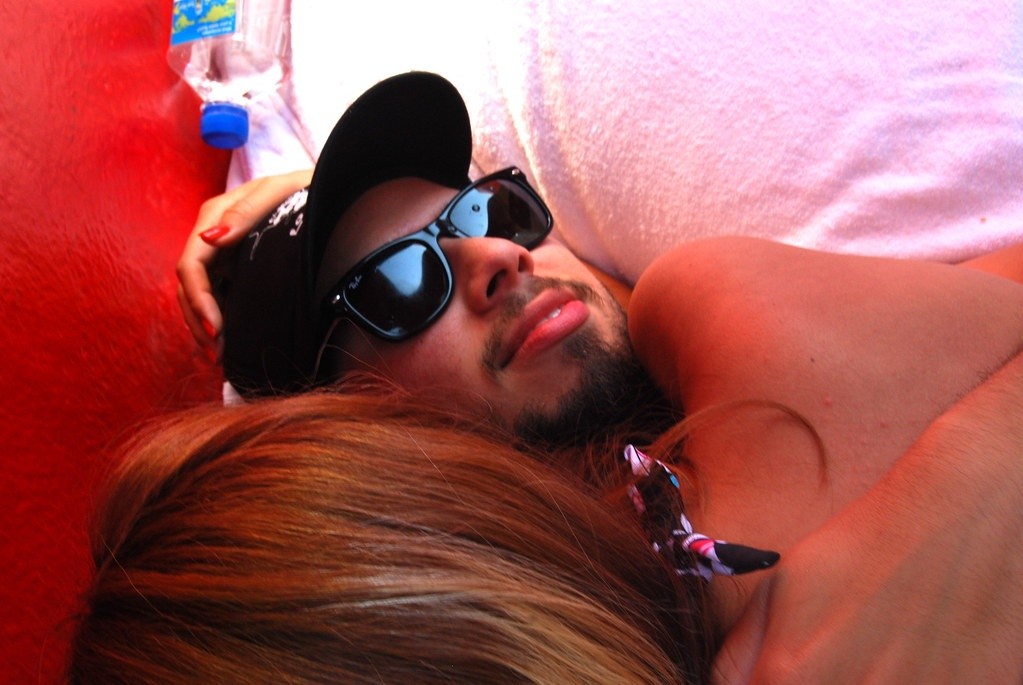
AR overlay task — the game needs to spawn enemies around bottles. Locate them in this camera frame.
[166,0,293,149]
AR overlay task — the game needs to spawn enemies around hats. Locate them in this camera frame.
[205,70,472,403]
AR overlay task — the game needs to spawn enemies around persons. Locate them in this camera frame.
[202,71,1023,685]
[69,167,1023,685]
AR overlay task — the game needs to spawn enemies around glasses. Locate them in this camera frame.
[310,164,553,385]
